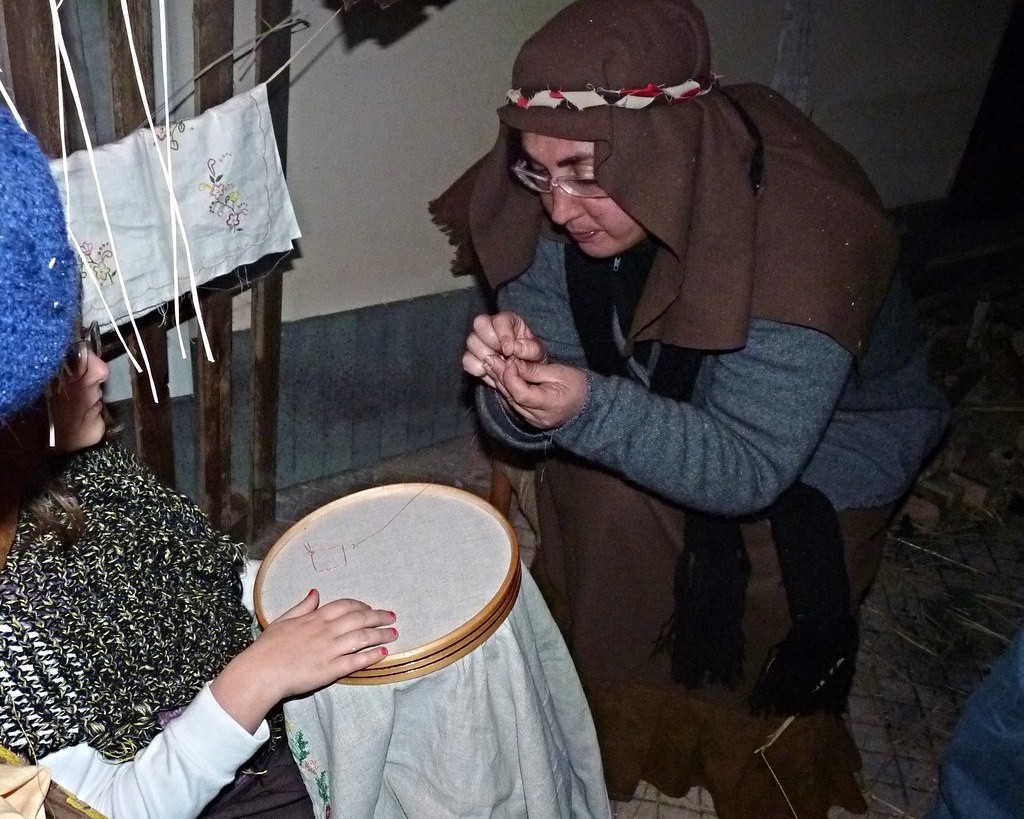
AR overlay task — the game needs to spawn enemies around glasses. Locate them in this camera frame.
[511,159,610,198]
[53,320,102,385]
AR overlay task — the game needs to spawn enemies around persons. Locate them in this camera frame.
[460,0,986,726]
[0,102,400,818]
